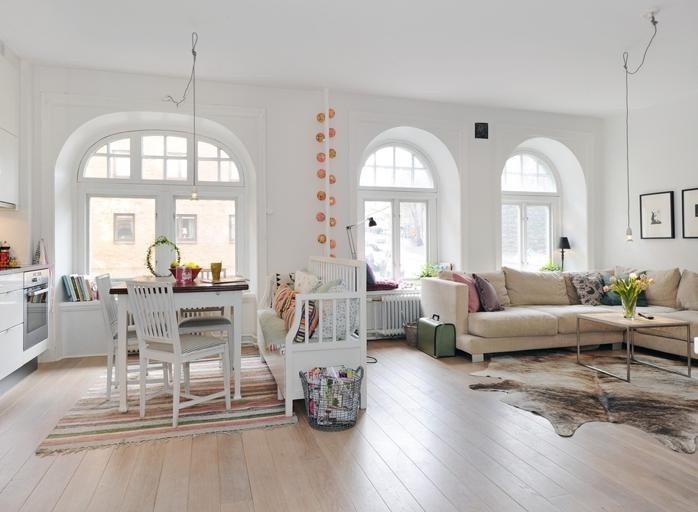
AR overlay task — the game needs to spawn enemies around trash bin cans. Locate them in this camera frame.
[299,366,364,432]
[403,322,418,348]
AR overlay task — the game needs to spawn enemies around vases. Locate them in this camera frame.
[617,294,639,320]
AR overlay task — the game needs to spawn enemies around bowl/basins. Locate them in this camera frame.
[168,268,203,281]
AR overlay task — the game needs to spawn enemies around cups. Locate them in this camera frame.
[175,266,193,284]
[211,262,222,281]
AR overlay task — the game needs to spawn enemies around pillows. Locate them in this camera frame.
[451,272,480,314]
[271,282,299,320]
[675,268,698,313]
[284,292,320,344]
[604,271,649,308]
[473,274,504,311]
[367,277,399,292]
[646,268,684,314]
[569,272,605,306]
[366,262,376,285]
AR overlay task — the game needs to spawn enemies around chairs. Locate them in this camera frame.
[125,278,232,428]
[178,267,232,394]
[94,272,173,402]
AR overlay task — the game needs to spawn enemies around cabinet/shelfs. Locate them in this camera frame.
[0,265,52,382]
[0,57,23,210]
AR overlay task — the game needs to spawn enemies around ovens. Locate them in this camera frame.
[24,267,49,351]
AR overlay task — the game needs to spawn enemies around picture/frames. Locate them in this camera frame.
[680,186,698,240]
[637,190,676,241]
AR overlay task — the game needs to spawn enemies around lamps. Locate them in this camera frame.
[556,233,572,274]
[163,31,201,199]
[344,217,378,260]
[623,5,659,244]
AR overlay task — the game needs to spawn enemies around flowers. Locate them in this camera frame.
[602,273,655,317]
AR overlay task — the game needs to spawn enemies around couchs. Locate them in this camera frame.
[257,254,368,416]
[421,276,698,364]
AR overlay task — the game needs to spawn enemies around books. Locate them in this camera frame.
[61,273,97,301]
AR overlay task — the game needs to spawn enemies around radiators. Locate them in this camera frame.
[378,293,421,337]
